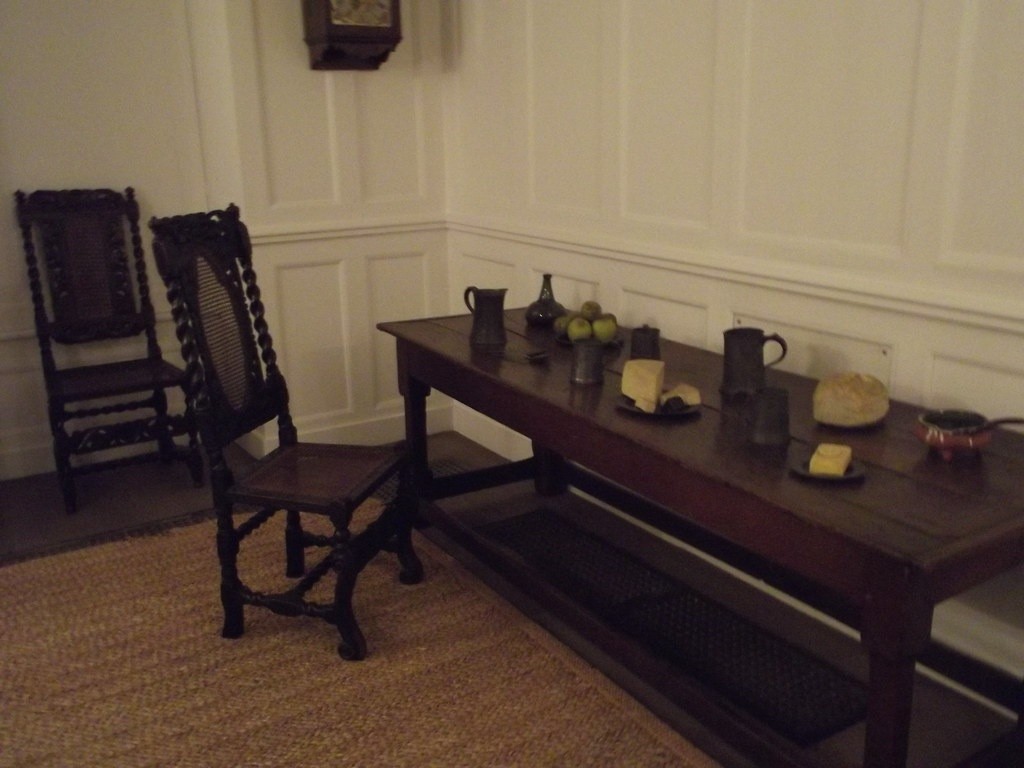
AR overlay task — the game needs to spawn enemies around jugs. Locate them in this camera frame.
[464,286,508,353]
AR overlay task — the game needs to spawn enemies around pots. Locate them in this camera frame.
[914,408,1024,450]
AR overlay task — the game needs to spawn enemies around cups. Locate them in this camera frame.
[630,324,661,360]
[570,338,622,386]
[719,327,788,396]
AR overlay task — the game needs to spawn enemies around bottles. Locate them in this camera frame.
[525,274,567,327]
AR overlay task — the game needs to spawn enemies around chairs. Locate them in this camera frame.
[147,202,423,660]
[12,187,201,513]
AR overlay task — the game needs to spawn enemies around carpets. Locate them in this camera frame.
[0,497,747,768]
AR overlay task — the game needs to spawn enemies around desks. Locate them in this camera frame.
[374,306,1024,768]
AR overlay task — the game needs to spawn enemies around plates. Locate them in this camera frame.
[555,332,622,348]
[791,459,866,481]
[615,388,699,416]
